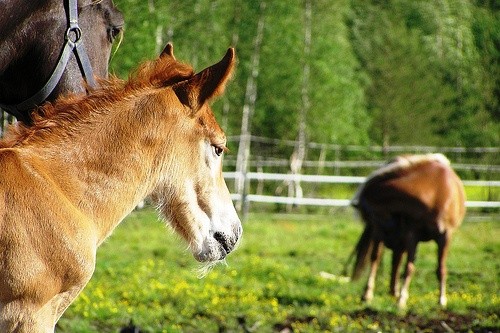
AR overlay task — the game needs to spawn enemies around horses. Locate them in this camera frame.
[344,153,467,311]
[0,0,244,333]
